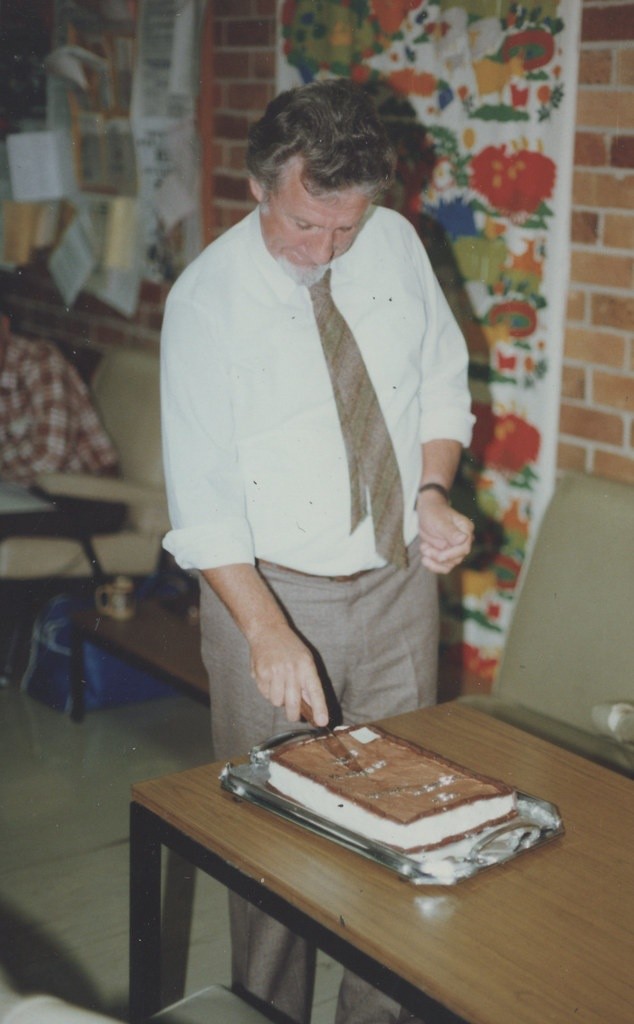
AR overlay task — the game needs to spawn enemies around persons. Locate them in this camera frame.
[0,304,129,677]
[161,81,477,1024]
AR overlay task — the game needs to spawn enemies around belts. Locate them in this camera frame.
[254,545,408,581]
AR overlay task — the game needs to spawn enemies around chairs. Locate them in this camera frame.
[1,350,171,710]
[69,597,210,720]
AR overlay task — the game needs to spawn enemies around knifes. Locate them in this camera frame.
[300,698,363,772]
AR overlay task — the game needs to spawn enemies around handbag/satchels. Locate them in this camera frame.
[20,580,178,715]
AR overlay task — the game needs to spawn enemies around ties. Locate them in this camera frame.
[307,270,410,571]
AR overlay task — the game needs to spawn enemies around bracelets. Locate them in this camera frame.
[420,483,452,505]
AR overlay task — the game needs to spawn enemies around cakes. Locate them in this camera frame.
[268,722,519,855]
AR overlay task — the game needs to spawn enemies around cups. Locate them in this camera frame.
[95,575,135,620]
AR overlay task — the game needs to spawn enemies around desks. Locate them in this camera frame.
[126,698,634,1024]
